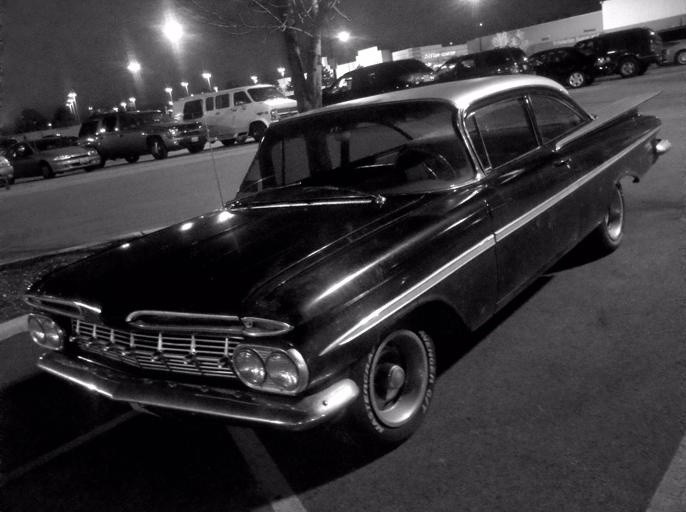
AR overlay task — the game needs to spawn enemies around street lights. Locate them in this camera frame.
[479,22,484,51]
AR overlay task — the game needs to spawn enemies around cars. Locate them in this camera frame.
[527,46,614,90]
[23,74,672,446]
[657,24,686,66]
[0,156,16,186]
[5,135,101,179]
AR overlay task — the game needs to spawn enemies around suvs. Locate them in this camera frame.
[318,58,440,105]
[174,83,298,154]
[436,47,533,82]
[78,108,209,164]
[571,27,666,79]
